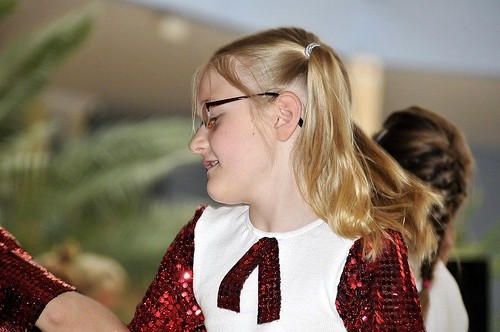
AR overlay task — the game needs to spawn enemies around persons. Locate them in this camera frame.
[129,27,437,332]
[0,225,129,332]
[372,106,471,332]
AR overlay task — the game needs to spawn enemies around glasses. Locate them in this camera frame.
[201,92,304,129]
